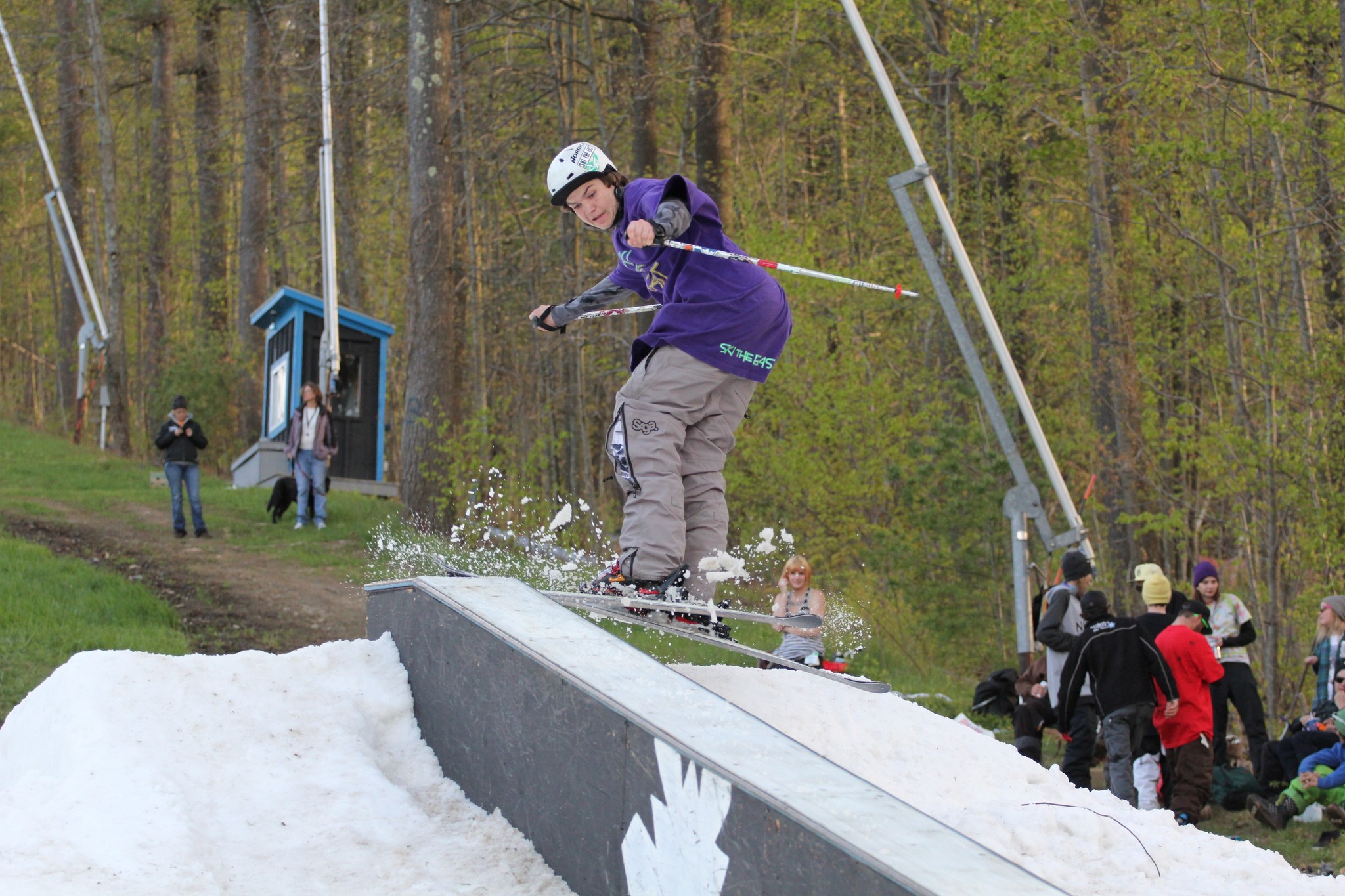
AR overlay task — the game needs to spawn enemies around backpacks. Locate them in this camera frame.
[1209,754,1263,810]
[974,669,1020,716]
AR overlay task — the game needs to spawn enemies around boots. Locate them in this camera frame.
[1246,793,1292,831]
[1327,800,1345,830]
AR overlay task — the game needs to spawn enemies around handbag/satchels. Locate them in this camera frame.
[1133,753,1161,809]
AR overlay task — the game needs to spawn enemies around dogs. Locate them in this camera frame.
[267,476,331,524]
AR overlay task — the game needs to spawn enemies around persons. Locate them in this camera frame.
[766,554,825,669]
[155,395,212,539]
[285,381,338,529]
[1012,550,1345,830]
[528,142,792,637]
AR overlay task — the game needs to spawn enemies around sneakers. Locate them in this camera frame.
[672,612,706,630]
[580,566,666,617]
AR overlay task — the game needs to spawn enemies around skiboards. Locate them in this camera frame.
[438,565,893,693]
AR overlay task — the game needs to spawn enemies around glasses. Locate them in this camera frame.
[1319,605,1331,614]
[1335,677,1345,683]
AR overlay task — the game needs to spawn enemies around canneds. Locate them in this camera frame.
[1210,641,1222,660]
[1036,680,1048,698]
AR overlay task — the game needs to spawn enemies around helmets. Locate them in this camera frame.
[547,143,617,206]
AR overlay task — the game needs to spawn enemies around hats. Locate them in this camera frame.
[1081,590,1107,620]
[1182,600,1213,635]
[1062,552,1092,582]
[1133,563,1163,581]
[1193,562,1218,588]
[1142,573,1172,605]
[1322,594,1345,621]
[174,396,187,409]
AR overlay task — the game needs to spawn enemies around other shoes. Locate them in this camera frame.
[317,521,328,530]
[295,522,303,529]
[175,530,186,538]
[198,530,212,538]
